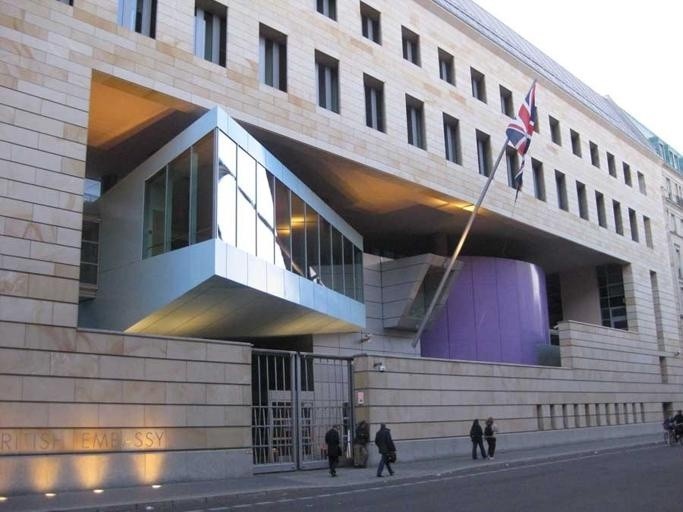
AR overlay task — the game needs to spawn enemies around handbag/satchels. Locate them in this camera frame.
[485,426,494,436]
[385,452,397,462]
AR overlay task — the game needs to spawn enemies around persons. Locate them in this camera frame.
[661,414,673,432]
[670,409,682,442]
[352,418,370,468]
[323,424,344,476]
[469,418,486,460]
[373,422,396,477]
[484,415,498,460]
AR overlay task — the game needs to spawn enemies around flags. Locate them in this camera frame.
[504,80,537,203]
